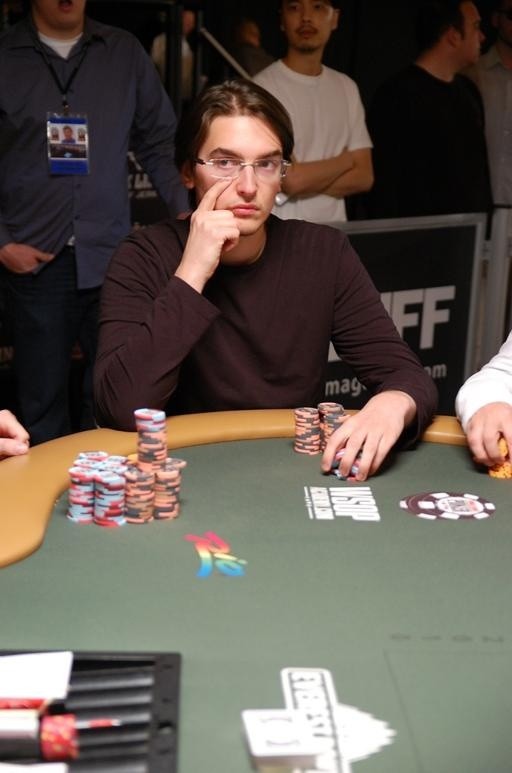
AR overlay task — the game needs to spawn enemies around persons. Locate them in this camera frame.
[1,409,30,459]
[92,80,441,481]
[228,20,275,82]
[455,332,511,469]
[150,9,196,99]
[356,1,497,243]
[251,1,376,223]
[61,126,75,143]
[1,0,196,448]
[463,0,511,207]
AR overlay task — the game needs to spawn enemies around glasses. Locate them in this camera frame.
[196,157,292,182]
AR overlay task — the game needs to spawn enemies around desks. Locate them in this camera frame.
[1,403,511,772]
